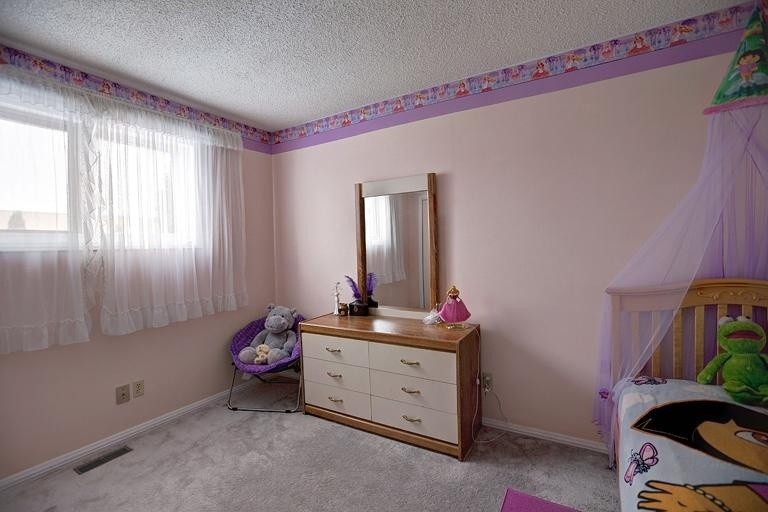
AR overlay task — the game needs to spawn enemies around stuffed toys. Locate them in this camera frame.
[697,315,768,406]
[254,344,269,364]
[238,303,298,365]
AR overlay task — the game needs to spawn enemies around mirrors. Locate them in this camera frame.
[349,170,442,314]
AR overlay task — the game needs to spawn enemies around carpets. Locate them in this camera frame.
[0,372,619,512]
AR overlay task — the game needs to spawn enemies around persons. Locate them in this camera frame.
[439,286,471,329]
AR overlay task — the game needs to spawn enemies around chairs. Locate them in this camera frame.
[227,306,308,415]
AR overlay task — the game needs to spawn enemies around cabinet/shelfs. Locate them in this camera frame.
[296,308,484,464]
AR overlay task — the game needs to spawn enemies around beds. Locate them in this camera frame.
[604,277,768,512]
